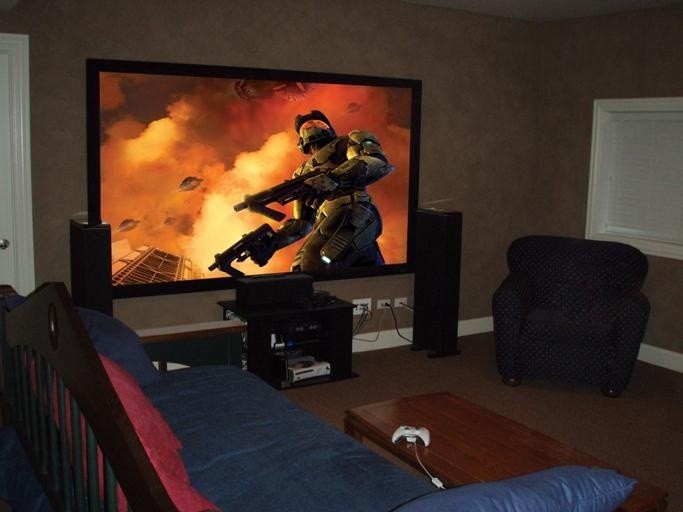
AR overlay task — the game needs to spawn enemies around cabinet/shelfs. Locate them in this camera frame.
[217,299,360,390]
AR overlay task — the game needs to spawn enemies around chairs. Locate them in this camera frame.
[492,235,652,398]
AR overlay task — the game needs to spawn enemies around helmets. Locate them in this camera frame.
[294,109,336,152]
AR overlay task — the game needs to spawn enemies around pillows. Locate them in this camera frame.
[22,351,220,512]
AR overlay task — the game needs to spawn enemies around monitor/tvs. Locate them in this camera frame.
[85,56,423,298]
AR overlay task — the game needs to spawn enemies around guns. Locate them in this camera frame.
[233,155,347,221]
[209,223,276,277]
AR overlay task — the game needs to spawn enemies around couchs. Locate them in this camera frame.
[0,281,639,512]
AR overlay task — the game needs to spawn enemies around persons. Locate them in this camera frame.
[244,120,392,272]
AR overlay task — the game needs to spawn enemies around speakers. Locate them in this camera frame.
[68,216,113,317]
[410,206,464,357]
